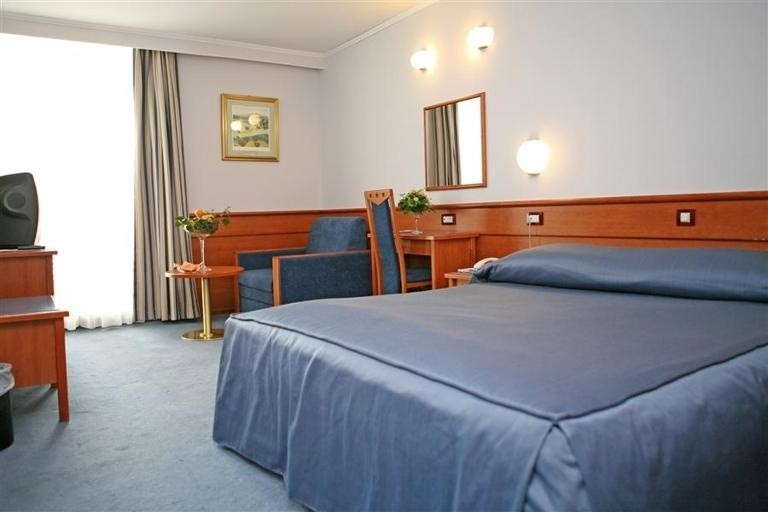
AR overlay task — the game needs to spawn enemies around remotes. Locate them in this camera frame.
[18,246,45,250]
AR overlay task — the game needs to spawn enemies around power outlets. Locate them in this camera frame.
[528,211,544,226]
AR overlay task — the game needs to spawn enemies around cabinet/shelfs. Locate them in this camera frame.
[0,246,62,389]
[0,294,72,423]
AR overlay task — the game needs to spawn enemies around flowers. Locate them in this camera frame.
[398,190,431,216]
[173,205,232,233]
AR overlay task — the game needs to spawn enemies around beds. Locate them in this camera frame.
[212,240,768,512]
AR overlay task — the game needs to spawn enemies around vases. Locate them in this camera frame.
[410,212,423,236]
[187,229,215,272]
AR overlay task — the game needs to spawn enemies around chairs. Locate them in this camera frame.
[363,188,431,296]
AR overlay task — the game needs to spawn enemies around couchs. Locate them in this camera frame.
[233,216,372,314]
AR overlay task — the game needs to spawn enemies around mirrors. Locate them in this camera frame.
[421,92,489,190]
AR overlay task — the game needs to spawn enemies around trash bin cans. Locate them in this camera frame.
[0,362,15,452]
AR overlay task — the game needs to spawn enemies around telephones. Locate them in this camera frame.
[472,257,499,272]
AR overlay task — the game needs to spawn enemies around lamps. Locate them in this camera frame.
[471,25,494,52]
[516,138,549,176]
[410,47,434,74]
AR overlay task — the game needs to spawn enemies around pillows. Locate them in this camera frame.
[472,243,767,304]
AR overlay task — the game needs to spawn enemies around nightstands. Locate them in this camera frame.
[442,271,472,288]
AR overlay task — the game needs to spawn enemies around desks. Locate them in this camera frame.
[163,266,246,340]
[366,230,478,291]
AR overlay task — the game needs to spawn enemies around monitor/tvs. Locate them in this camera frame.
[0,173,38,250]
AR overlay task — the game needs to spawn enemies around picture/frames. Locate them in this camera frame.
[221,93,280,163]
